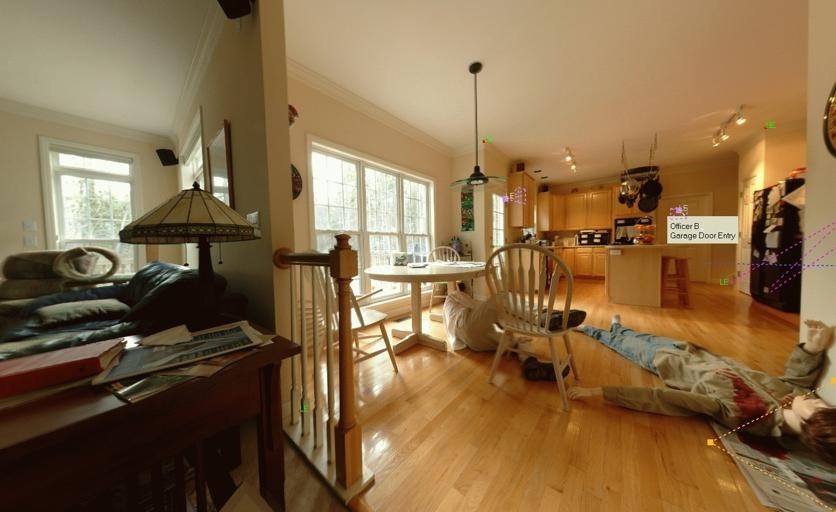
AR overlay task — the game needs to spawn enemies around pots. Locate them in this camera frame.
[617,177,663,212]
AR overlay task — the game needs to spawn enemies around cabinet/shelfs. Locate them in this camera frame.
[507,246,663,308]
[535,182,656,231]
[507,171,537,230]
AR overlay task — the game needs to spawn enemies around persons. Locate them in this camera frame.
[441,282,588,381]
[564,312,835,469]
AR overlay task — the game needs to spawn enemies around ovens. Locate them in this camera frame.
[615,218,652,243]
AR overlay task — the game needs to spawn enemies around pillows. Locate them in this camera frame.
[33,294,132,330]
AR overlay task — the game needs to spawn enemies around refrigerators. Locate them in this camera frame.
[749,177,805,313]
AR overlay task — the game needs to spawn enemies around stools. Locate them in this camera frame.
[661,253,694,309]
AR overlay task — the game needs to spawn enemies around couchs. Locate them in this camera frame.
[2,259,248,365]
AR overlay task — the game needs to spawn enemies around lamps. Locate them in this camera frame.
[447,61,511,195]
[711,104,748,147]
[119,179,261,320]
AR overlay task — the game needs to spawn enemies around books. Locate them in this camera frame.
[90,320,279,405]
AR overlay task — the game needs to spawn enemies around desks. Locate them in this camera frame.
[0,311,303,511]
[365,258,498,356]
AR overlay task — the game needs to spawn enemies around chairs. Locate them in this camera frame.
[300,249,400,375]
[484,244,587,413]
[427,245,464,311]
[0,245,102,335]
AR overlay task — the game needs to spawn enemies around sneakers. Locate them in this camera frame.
[522,357,569,380]
[544,310,586,332]
